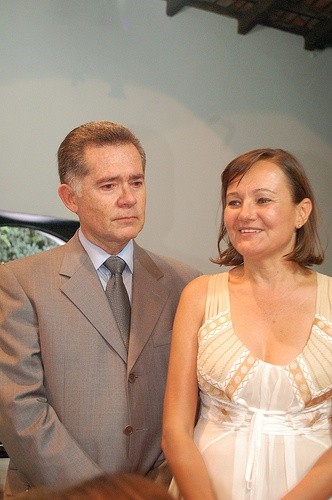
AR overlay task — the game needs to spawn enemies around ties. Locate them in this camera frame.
[101,254,132,352]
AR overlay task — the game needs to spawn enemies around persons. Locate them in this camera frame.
[15,469,179,500]
[0,119,206,495]
[159,147,332,500]
